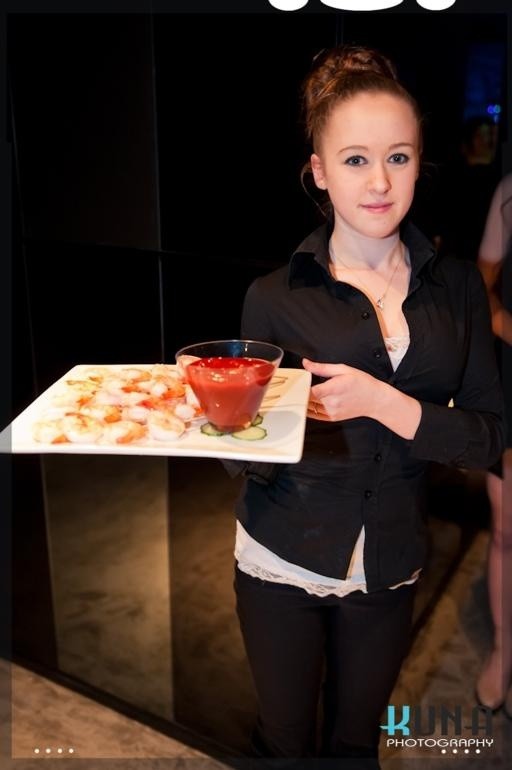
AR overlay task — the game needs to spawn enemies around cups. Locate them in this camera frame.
[172,337,287,435]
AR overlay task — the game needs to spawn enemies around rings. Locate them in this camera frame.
[314,403,318,414]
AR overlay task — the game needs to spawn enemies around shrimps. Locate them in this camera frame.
[31,365,203,444]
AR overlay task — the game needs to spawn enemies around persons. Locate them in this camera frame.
[233,41,510,770]
[477,174,512,709]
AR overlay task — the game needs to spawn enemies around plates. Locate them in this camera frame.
[0,362,313,467]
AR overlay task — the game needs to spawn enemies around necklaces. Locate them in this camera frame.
[353,255,403,309]
